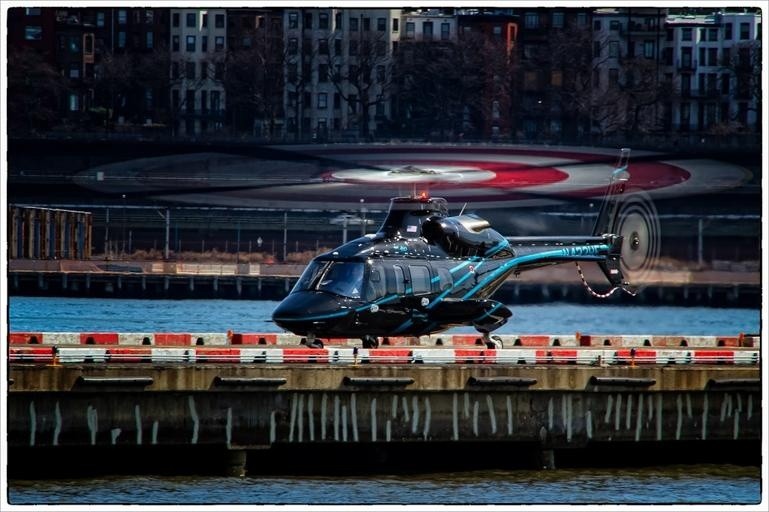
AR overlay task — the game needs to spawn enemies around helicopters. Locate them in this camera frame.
[70,142,755,347]
